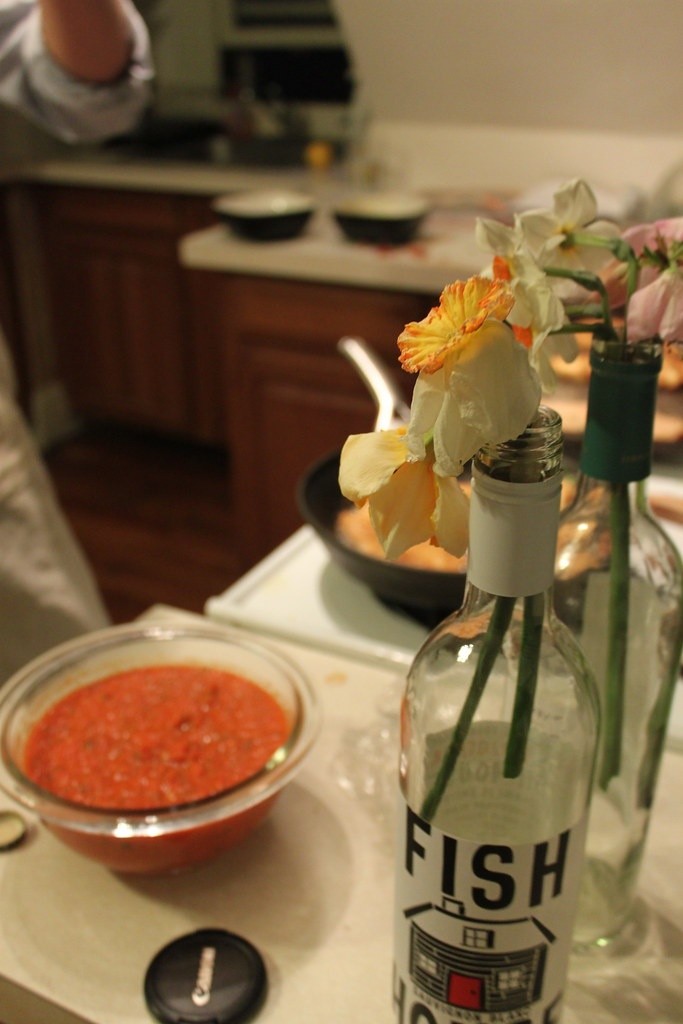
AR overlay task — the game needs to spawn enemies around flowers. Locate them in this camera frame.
[339,259,559,819]
[475,175,683,792]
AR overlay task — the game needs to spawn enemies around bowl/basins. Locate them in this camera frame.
[212,193,314,242]
[2,623,322,879]
[329,198,427,245]
[293,443,466,628]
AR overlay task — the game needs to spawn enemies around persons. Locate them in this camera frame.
[0,0,153,688]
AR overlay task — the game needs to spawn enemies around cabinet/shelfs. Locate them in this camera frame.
[34,181,227,443]
[217,269,442,577]
